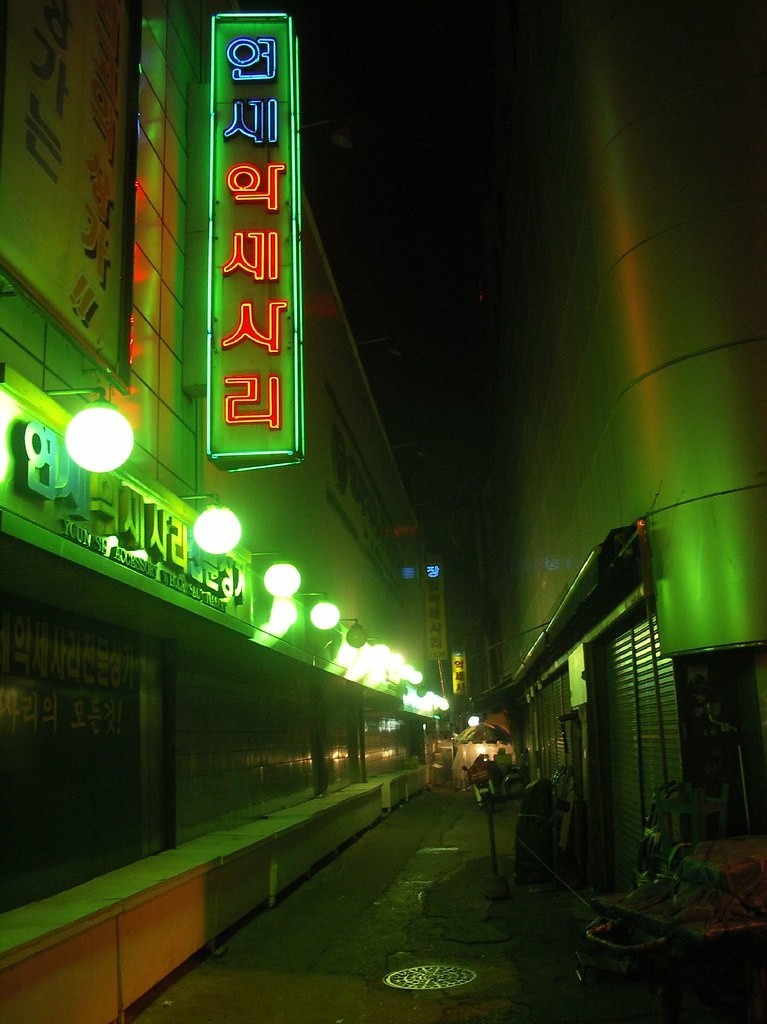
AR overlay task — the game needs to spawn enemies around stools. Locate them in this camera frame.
[654,783,730,857]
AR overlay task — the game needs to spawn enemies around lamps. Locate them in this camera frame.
[339,618,367,648]
[251,552,302,597]
[298,592,340,630]
[44,386,134,473]
[178,493,242,554]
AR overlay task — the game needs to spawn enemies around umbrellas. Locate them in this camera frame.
[454,721,513,746]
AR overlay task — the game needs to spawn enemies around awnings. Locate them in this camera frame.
[469,678,519,713]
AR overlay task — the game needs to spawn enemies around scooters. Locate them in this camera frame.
[462,764,496,814]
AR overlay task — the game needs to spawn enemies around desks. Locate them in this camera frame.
[590,892,767,1024]
[0,763,431,1024]
